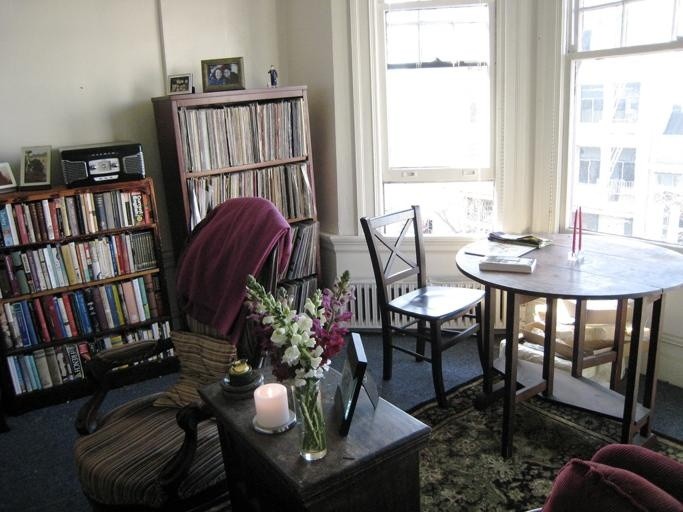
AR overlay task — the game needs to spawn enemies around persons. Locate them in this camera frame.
[174,79,188,92]
[267,63,279,87]
[208,64,239,86]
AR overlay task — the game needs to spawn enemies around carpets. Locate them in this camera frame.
[404,370,683,512]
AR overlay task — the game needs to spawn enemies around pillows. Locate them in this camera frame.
[544,458,682,512]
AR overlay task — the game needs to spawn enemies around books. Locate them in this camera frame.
[479,254,537,274]
[0,190,176,395]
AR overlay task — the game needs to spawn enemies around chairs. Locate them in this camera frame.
[540,441,683,512]
[67,196,292,512]
[360,203,488,409]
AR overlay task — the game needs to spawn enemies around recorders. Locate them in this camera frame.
[58,141,147,189]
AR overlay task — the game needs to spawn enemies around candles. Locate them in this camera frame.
[253,383,290,428]
[571,209,578,253]
[579,205,583,251]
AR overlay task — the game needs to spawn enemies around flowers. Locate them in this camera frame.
[241,267,357,452]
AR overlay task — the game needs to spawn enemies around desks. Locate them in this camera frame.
[455,235,683,460]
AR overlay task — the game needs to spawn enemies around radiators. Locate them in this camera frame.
[334,280,507,332]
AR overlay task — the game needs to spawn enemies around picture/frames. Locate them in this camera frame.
[20,144,52,187]
[0,162,17,190]
[167,72,193,95]
[200,57,246,93]
[334,330,381,437]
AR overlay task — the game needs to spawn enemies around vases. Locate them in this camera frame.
[294,380,326,461]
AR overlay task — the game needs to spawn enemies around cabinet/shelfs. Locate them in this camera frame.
[0,175,176,417]
[201,357,430,511]
[151,85,323,353]
